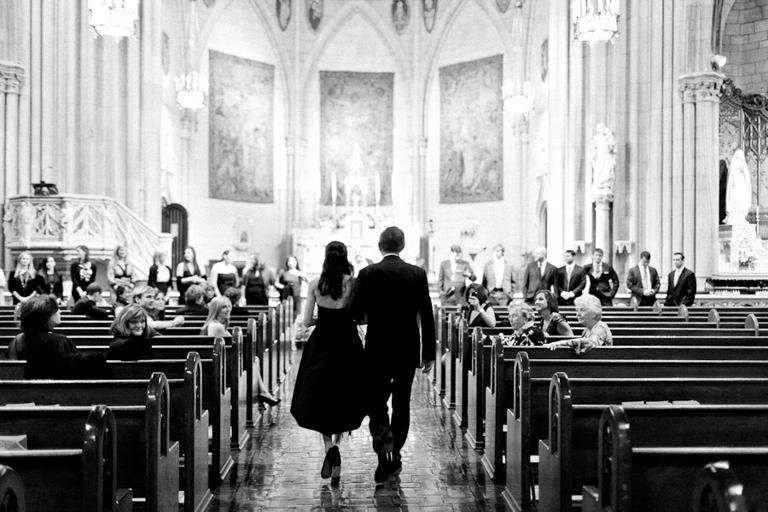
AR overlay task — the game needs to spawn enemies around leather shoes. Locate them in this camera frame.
[375,451,401,483]
[321,446,341,486]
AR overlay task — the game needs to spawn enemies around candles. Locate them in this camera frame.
[374,171,382,202]
[330,171,337,202]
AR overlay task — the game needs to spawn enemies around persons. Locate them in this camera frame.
[275,256,308,352]
[665,253,694,307]
[627,251,661,307]
[348,226,435,482]
[290,240,368,487]
[438,244,619,352]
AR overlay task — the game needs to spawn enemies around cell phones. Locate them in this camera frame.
[472,292,477,297]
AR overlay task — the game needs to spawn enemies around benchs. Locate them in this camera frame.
[2,289,297,512]
[434,296,768,512]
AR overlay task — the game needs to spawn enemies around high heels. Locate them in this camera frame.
[259,395,281,407]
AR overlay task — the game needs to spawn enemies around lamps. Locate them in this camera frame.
[85,0,142,42]
[708,53,728,72]
[568,0,622,46]
